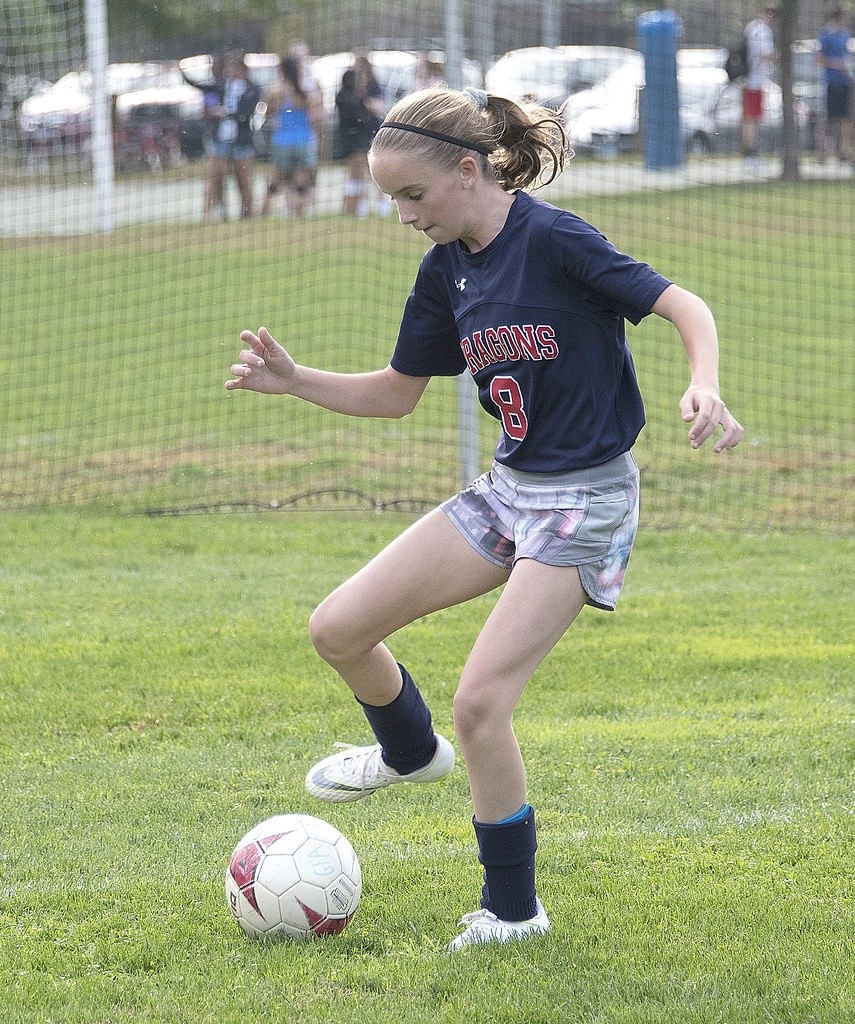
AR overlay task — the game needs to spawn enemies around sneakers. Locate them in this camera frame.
[441,897,555,951]
[305,733,455,806]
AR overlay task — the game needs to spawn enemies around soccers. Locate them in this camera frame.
[224,813,363,940]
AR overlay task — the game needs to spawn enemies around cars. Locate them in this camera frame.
[16,38,827,164]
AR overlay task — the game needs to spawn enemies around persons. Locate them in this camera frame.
[821,6,855,164]
[335,52,449,222]
[225,89,745,950]
[743,5,783,155]
[181,39,323,222]
